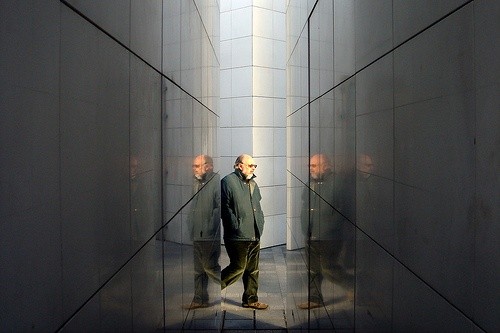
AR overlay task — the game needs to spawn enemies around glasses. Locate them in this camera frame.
[308,164,323,168]
[362,164,373,167]
[239,161,257,168]
[194,162,208,168]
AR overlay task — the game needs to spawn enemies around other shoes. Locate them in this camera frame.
[298,302,322,309]
[243,301,268,308]
[182,303,208,309]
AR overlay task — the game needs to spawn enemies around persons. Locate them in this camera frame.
[343,152,374,258]
[297,151,353,309]
[218,153,269,310]
[179,154,220,311]
